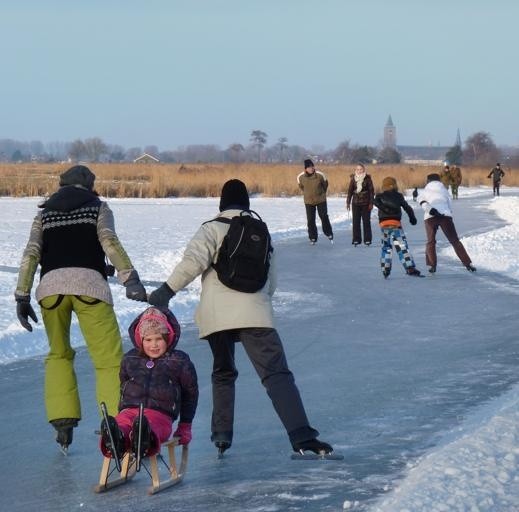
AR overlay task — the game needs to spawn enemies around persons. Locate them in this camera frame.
[99,306,199,459]
[450,165,462,200]
[440,166,450,190]
[149,180,334,456]
[15,165,147,448]
[488,163,505,196]
[372,177,420,277]
[347,163,374,245]
[418,174,476,274]
[297,159,334,243]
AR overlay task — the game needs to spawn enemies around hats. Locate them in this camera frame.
[220,180,249,211]
[61,165,95,188]
[134,308,174,347]
[383,177,397,191]
[428,174,440,181]
[304,159,313,169]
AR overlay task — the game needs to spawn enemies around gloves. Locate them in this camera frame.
[149,282,175,307]
[173,422,192,444]
[14,295,37,331]
[124,270,147,301]
[430,208,444,217]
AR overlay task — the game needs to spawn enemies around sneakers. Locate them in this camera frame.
[294,439,333,454]
[57,426,72,444]
[133,416,150,449]
[467,264,476,271]
[383,271,389,275]
[213,431,232,448]
[429,266,435,272]
[408,268,419,274]
[101,416,119,450]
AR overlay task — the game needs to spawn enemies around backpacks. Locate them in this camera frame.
[202,210,273,293]
